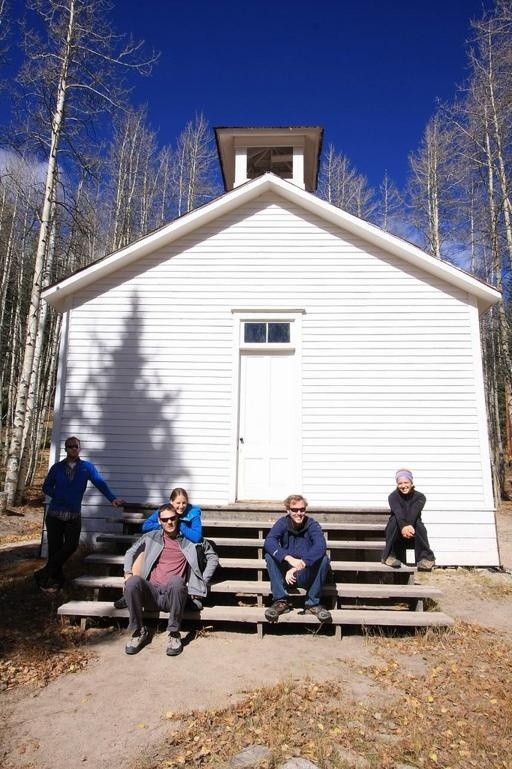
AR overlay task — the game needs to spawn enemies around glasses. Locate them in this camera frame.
[289,507,306,512]
[67,443,79,449]
[160,515,177,522]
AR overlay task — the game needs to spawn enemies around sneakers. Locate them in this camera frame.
[381,556,401,567]
[34,569,48,592]
[48,577,62,592]
[126,631,148,655]
[304,604,330,620]
[114,596,127,609]
[265,600,293,618]
[188,597,203,611]
[167,632,183,656]
[416,558,435,569]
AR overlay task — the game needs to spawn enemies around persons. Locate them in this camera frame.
[125,502,206,658]
[115,487,221,613]
[32,438,125,595]
[262,492,346,621]
[379,469,438,575]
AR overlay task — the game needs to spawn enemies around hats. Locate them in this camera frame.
[395,470,413,484]
